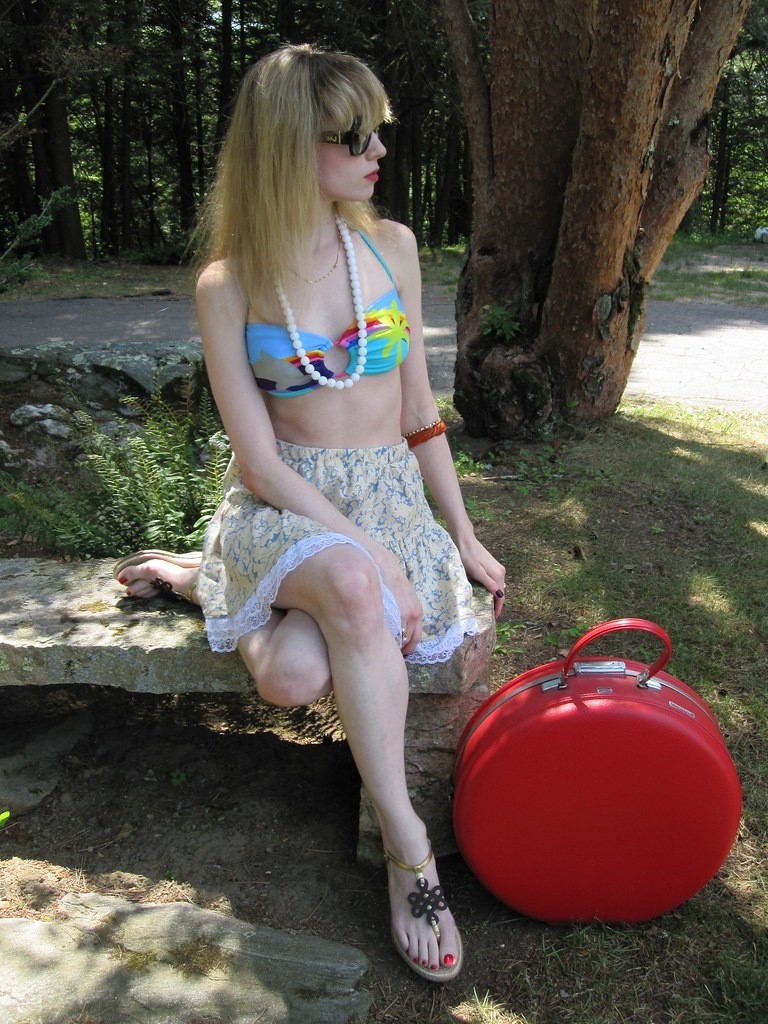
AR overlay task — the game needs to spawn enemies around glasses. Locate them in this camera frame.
[307,115,377,157]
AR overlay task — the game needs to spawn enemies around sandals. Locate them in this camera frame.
[384,838,463,982]
[113,550,203,603]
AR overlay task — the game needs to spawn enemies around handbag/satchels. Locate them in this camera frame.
[448,618,742,923]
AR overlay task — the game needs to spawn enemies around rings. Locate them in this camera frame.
[400,626,407,641]
[504,582,507,590]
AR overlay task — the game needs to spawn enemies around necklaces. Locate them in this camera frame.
[264,206,368,388]
[283,234,343,284]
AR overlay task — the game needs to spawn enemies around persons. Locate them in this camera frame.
[113,44,507,982]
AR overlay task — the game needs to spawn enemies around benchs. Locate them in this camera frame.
[0,558,505,867]
